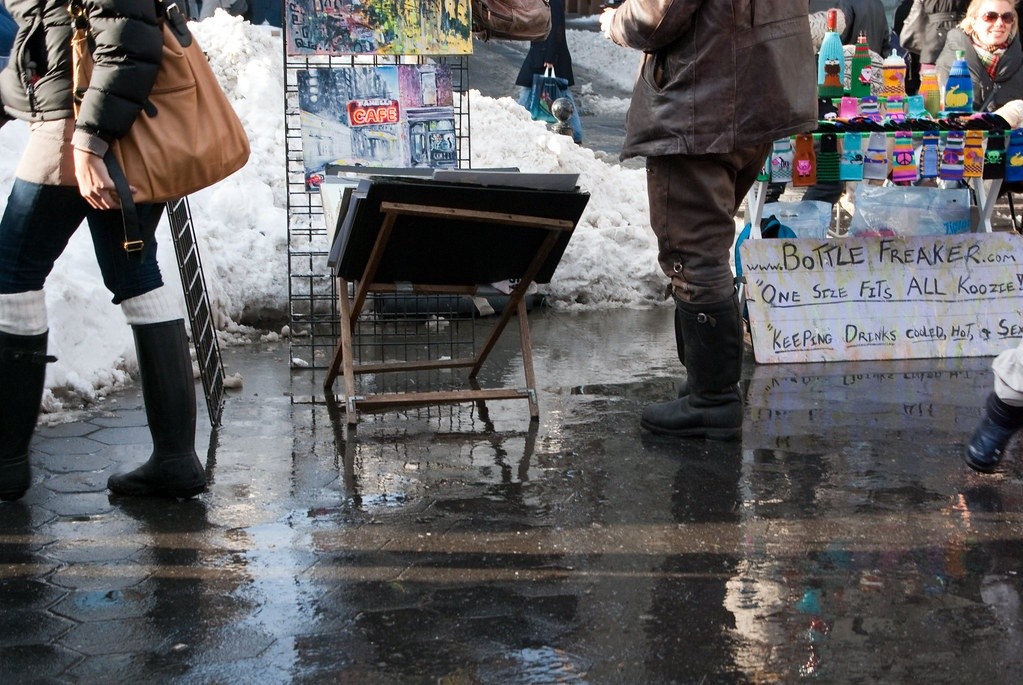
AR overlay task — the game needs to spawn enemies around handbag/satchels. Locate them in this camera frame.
[68,1,250,211]
[471,0,552,42]
[531,65,581,143]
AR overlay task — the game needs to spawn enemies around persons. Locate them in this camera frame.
[963,335,1023,473]
[808,0,972,97]
[935,0,1023,113]
[0,0,208,502]
[600,0,819,440]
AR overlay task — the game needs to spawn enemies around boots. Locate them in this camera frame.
[1,328,57,500]
[107,318,207,497]
[641,285,743,440]
[965,391,1022,472]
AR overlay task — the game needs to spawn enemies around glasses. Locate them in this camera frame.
[978,11,1016,25]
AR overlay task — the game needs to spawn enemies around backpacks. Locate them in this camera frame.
[736,215,797,323]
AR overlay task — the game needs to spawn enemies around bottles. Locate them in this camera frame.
[756,95,1023,187]
[944,50,974,114]
[882,47,906,98]
[851,31,871,98]
[919,63,940,118]
[818,8,845,97]
